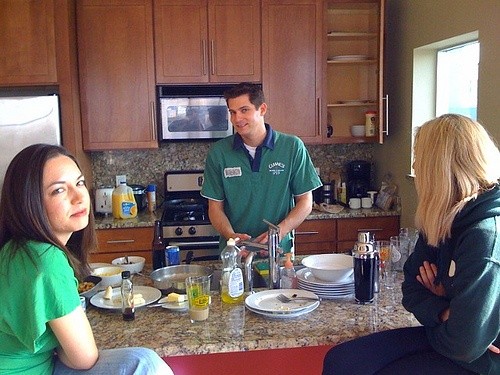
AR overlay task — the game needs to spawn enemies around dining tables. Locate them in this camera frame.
[82,255,424,375]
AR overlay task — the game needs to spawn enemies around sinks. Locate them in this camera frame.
[207,267,269,293]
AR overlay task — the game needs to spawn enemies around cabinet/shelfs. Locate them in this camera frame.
[295,216,399,254]
[88,227,153,264]
[75,0,389,152]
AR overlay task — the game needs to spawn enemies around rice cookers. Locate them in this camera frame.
[128,184,147,210]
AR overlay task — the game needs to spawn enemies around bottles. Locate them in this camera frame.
[152,219,166,267]
[220,238,244,303]
[352,231,379,305]
[111,181,137,220]
[144,184,156,201]
[120,271,135,321]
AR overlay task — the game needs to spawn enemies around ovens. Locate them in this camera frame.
[158,239,220,262]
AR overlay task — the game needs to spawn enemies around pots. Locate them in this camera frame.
[145,265,217,294]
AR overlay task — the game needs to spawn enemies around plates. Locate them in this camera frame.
[88,286,162,309]
[86,261,111,274]
[337,100,370,104]
[327,54,371,61]
[157,268,354,319]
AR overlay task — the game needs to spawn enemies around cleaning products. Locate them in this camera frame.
[219,236,244,305]
[281,253,299,289]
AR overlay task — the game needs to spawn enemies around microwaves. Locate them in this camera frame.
[157,86,239,140]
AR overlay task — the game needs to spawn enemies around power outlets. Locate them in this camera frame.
[116,175,126,187]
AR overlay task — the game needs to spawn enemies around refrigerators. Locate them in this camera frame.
[0,92,60,196]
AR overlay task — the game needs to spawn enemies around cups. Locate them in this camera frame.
[349,191,378,209]
[323,183,331,204]
[374,240,393,286]
[391,228,420,270]
[185,275,211,322]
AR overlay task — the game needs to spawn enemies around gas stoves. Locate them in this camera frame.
[160,170,221,238]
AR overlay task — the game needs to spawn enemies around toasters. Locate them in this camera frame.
[95,185,115,216]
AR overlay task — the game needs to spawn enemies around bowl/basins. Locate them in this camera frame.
[78,255,145,307]
[301,254,355,282]
[352,125,365,137]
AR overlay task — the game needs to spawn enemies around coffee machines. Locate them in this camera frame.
[344,160,371,206]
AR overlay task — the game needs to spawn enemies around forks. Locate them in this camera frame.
[276,293,322,303]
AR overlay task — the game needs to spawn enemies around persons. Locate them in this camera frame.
[0,144,173,375]
[322,113,500,375]
[200,80,323,262]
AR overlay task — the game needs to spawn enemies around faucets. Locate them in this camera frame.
[235,219,280,290]
[243,251,257,292]
